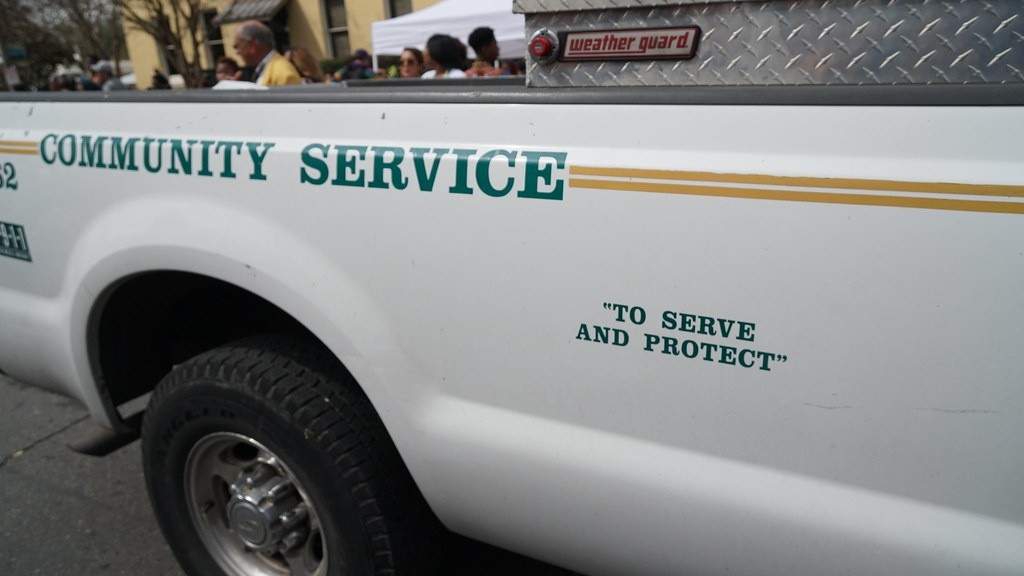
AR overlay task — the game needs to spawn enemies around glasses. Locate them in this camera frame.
[397,59,415,66]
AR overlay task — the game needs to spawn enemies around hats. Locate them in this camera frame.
[355,49,367,59]
[88,59,112,73]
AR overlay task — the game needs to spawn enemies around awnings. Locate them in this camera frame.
[210,0,286,25]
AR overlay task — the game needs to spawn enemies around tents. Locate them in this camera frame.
[372,0,526,74]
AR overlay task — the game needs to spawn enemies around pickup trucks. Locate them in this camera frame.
[1,2,1024,576]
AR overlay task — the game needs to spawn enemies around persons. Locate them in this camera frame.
[152,69,170,88]
[203,20,323,90]
[328,25,524,79]
[13,59,127,92]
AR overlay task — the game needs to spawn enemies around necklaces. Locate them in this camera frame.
[472,62,487,64]
